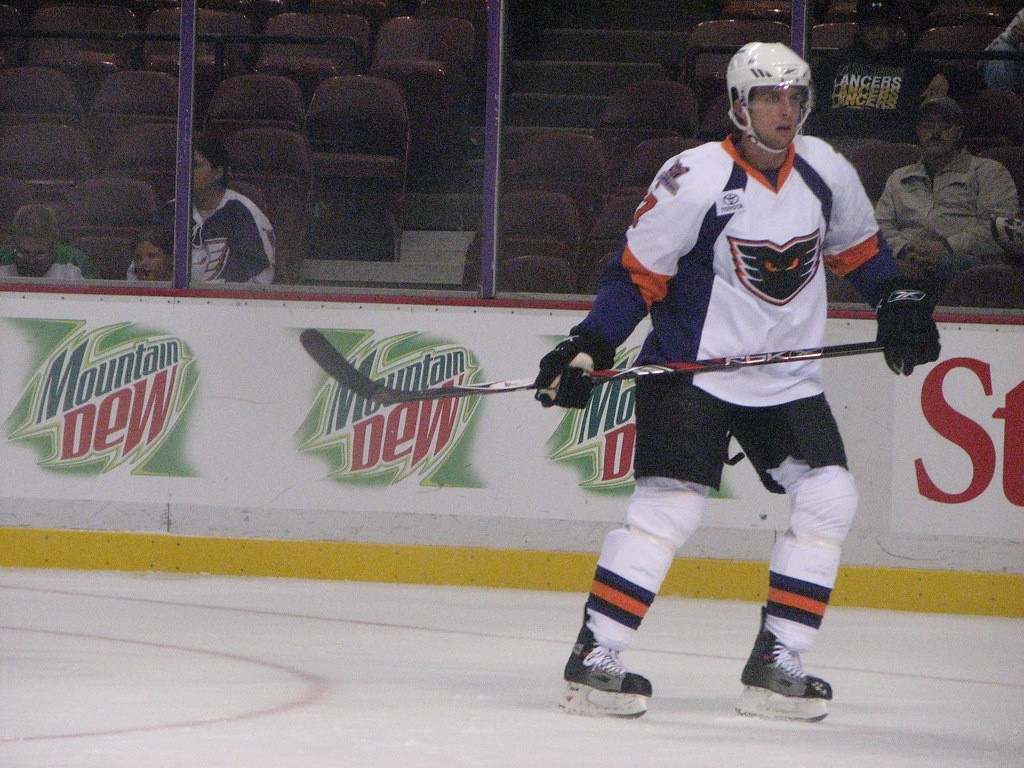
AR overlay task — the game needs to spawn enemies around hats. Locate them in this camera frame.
[916,95,964,128]
[856,0,898,25]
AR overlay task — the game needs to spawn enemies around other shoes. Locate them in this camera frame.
[990,214,1024,258]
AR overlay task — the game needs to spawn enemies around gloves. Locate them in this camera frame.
[533,326,614,410]
[874,279,941,376]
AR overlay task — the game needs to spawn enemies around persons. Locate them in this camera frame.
[0,205,101,278]
[802,0,1024,308]
[533,42,942,698]
[128,136,274,287]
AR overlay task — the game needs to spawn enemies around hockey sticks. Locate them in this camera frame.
[299,328,888,401]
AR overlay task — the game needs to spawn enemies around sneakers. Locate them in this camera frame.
[740,605,832,700]
[562,603,653,699]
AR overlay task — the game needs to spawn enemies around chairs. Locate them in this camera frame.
[0,0,1024,320]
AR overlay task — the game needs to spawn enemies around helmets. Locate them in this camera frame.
[726,41,815,113]
